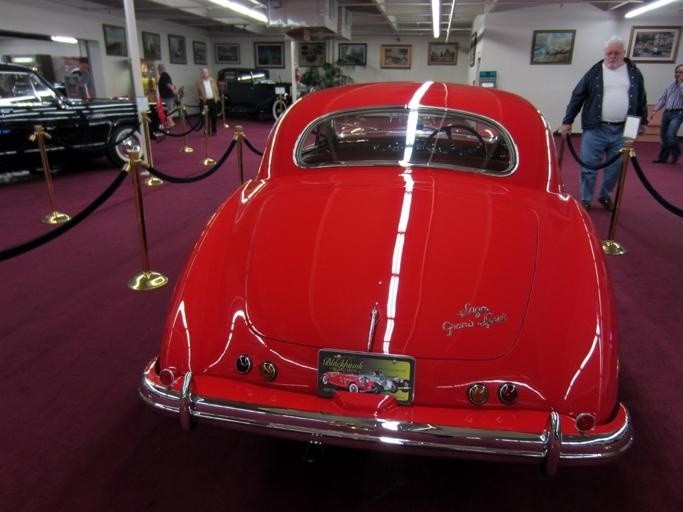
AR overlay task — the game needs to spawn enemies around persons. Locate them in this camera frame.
[157,63,178,128]
[559,37,649,213]
[193,67,220,137]
[648,65,683,164]
[70,62,96,98]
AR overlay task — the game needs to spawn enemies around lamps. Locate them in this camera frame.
[625,0,675,18]
[430,0,440,39]
[210,0,268,23]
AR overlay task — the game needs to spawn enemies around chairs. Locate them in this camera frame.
[636,104,665,142]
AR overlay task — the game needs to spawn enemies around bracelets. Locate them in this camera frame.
[652,109,658,113]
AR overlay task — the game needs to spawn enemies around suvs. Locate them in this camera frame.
[215,66,295,125]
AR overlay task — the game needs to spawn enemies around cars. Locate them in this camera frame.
[139,81,634,465]
[0,62,145,176]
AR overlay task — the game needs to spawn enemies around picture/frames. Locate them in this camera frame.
[142,29,162,61]
[193,38,208,67]
[253,40,285,68]
[169,34,186,65]
[103,26,129,60]
[626,25,682,63]
[212,43,242,65]
[379,43,412,70]
[428,40,458,67]
[338,40,368,67]
[301,43,326,67]
[531,28,576,64]
